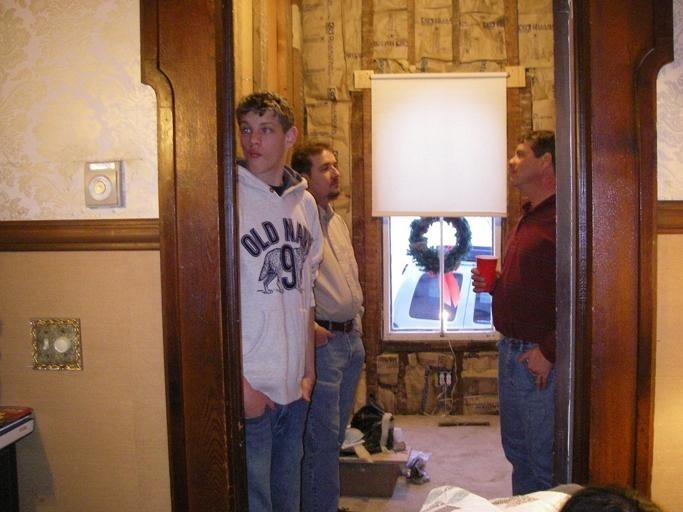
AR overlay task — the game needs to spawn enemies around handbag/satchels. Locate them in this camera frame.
[349,394,395,454]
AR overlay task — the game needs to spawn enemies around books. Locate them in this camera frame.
[0,404,35,448]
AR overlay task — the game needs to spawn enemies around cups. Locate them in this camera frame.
[474,255,498,292]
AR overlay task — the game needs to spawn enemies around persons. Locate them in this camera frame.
[290,142,369,511]
[468,130,556,496]
[233,89,318,511]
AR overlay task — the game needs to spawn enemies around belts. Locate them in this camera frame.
[314,319,355,333]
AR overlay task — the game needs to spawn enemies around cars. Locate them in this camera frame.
[389,243,494,331]
